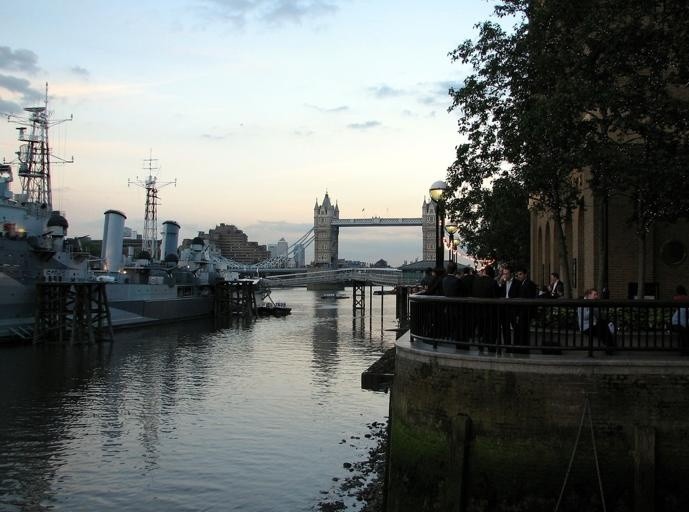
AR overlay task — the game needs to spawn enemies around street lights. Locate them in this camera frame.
[443,217,457,278]
[426,179,451,297]
[448,235,461,278]
[473,261,477,271]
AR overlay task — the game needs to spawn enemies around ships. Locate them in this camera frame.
[0,160,274,342]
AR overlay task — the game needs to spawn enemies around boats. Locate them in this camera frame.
[250,300,292,318]
[319,292,348,299]
[374,290,395,295]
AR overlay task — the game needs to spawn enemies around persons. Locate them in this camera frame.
[576,288,622,358]
[419,260,563,357]
[669,285,689,348]
